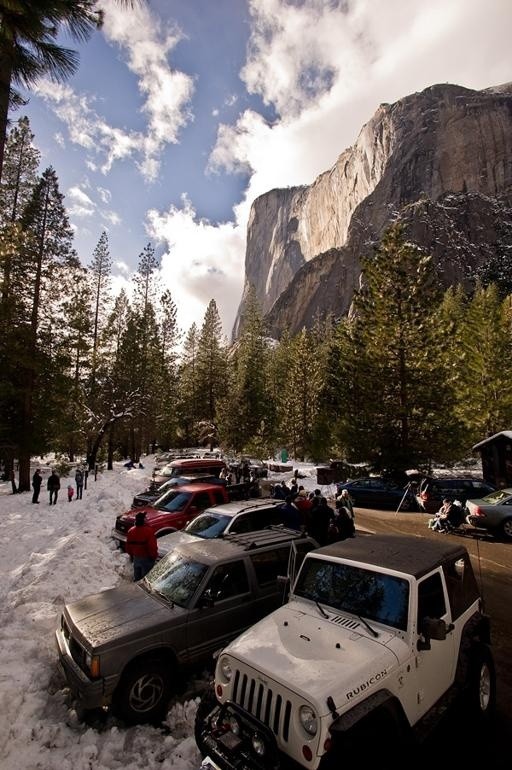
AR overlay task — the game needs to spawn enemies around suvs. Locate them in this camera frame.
[198,534,495,767]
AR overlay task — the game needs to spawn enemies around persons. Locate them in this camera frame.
[67,485,74,502]
[125,511,158,582]
[218,463,356,545]
[439,500,465,534]
[32,470,42,504]
[427,498,448,531]
[75,469,83,500]
[47,471,60,505]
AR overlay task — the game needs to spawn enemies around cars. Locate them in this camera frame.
[339,477,511,537]
